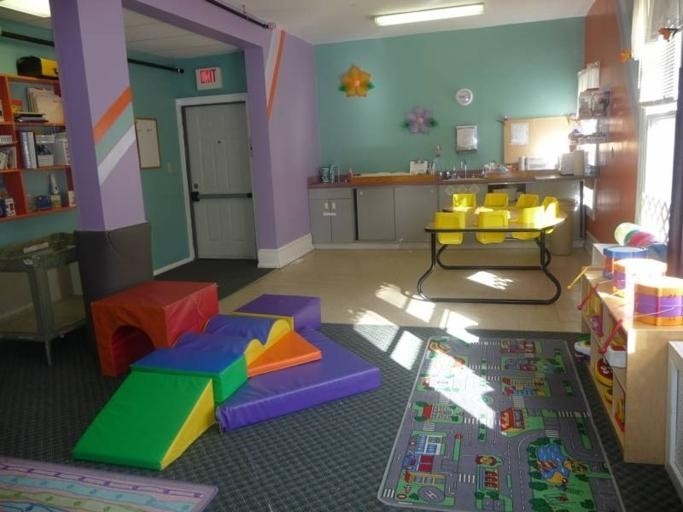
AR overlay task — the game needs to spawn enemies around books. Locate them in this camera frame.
[0,86,71,170]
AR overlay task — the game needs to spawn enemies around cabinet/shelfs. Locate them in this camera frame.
[0,74,77,225]
[589,243,621,265]
[437,183,488,250]
[349,176,438,250]
[579,267,683,466]
[662,341,683,498]
[0,241,88,367]
[525,179,584,249]
[576,91,610,142]
[307,181,356,250]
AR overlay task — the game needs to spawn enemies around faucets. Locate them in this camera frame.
[460,159,468,177]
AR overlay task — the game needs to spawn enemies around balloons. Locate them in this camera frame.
[613,222,657,246]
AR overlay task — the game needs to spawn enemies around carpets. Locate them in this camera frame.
[154,259,276,300]
[0,321,682,512]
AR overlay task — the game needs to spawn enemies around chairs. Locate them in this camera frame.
[416,192,569,305]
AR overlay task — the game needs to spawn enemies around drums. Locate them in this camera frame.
[603,244,683,328]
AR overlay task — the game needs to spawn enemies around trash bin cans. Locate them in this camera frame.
[544,197,576,256]
